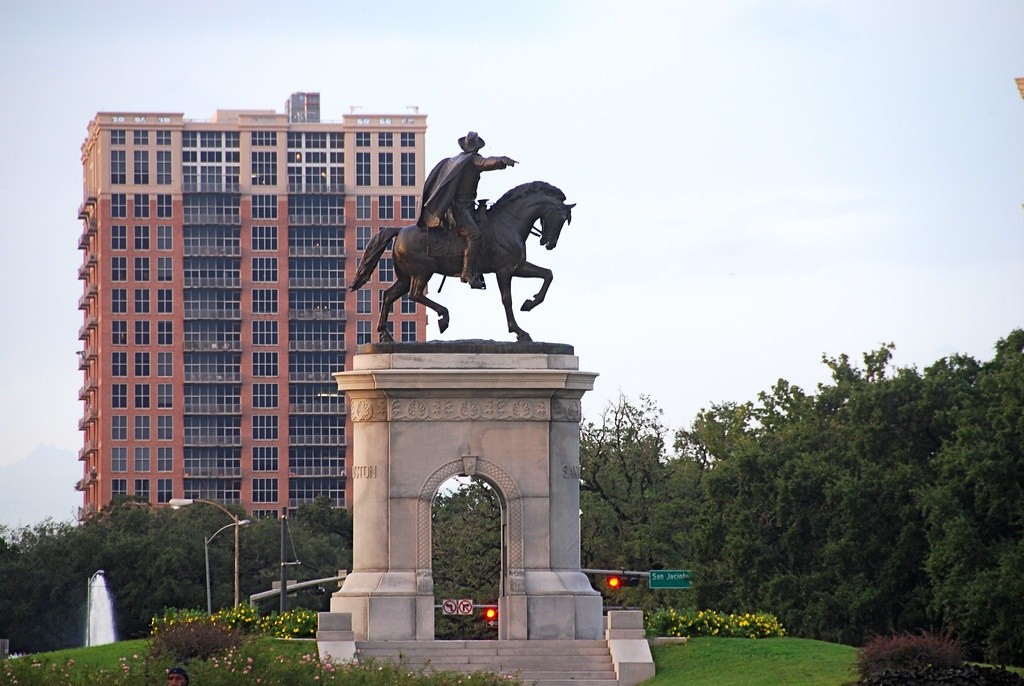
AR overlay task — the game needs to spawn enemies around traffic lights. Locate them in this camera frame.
[605,575,639,589]
[485,607,497,623]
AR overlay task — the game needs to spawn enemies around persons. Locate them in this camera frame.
[416,132,520,289]
[168,666,189,686]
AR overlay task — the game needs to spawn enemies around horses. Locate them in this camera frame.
[348,180,577,344]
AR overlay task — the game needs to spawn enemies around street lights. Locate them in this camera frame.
[204,518,251,617]
[169,497,240,615]
